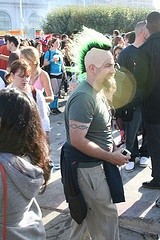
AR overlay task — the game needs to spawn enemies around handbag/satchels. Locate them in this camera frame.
[42,65,51,74]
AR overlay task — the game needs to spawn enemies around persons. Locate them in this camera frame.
[138,11,160,189]
[58,27,131,240]
[0,87,46,240]
[115,19,151,171]
[0,34,55,193]
[43,37,67,115]
[105,29,136,149]
[60,29,81,99]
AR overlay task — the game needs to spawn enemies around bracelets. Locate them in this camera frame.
[63,70,66,73]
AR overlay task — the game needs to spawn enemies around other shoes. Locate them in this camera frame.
[142,178,159,189]
[124,162,135,172]
[49,108,57,115]
[140,156,149,168]
[155,197,160,208]
[56,109,62,114]
